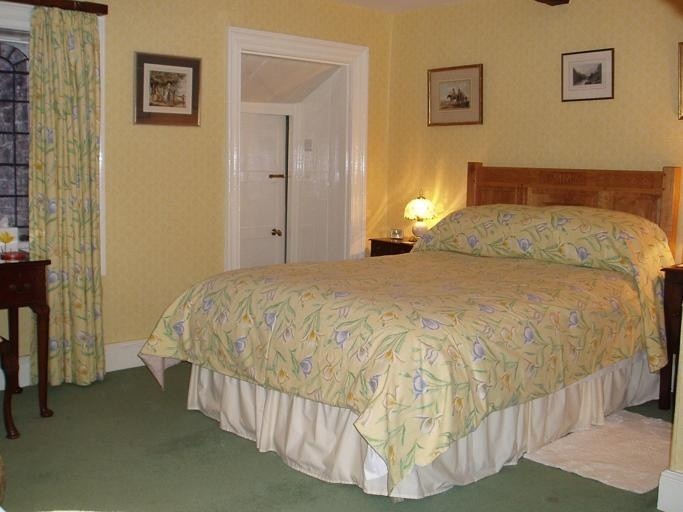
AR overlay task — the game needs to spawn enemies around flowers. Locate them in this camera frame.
[0,232,15,252]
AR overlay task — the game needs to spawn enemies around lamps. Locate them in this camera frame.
[403,186,438,241]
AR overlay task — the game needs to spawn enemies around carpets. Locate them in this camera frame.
[523,409,673,496]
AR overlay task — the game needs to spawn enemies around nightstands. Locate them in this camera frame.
[368,238,415,256]
[659,262,682,408]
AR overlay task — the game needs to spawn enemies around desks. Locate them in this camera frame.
[0,257,54,417]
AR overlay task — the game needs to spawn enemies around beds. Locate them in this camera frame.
[186,162,682,489]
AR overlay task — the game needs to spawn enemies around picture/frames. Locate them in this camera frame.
[427,63,482,126]
[561,48,614,102]
[133,51,201,128]
